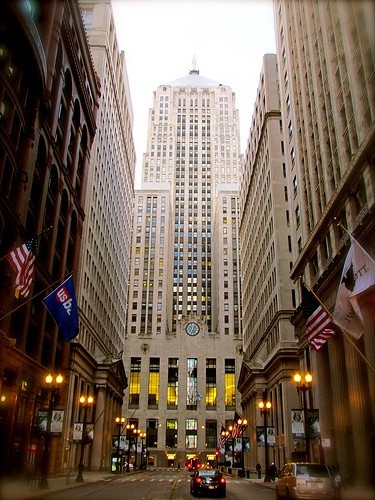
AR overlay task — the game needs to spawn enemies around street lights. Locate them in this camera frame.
[126,424,135,472]
[259,401,272,483]
[293,371,313,463]
[133,429,141,471]
[222,431,230,467]
[140,433,147,470]
[75,395,94,482]
[115,416,125,474]
[38,373,64,489]
[228,425,237,467]
[237,419,248,478]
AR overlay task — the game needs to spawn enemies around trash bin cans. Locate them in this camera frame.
[247,470,250,478]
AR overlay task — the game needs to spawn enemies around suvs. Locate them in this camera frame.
[273,462,341,500]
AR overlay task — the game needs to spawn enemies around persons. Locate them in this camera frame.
[292,412,304,433]
[255,462,262,471]
[270,462,277,482]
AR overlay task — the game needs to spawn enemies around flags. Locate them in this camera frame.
[331,236,375,340]
[42,275,79,344]
[5,235,41,302]
[298,282,340,354]
[219,425,231,445]
[232,412,247,438]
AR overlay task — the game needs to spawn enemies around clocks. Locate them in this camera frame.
[185,322,200,337]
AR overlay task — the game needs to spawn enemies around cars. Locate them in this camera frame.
[189,469,227,497]
